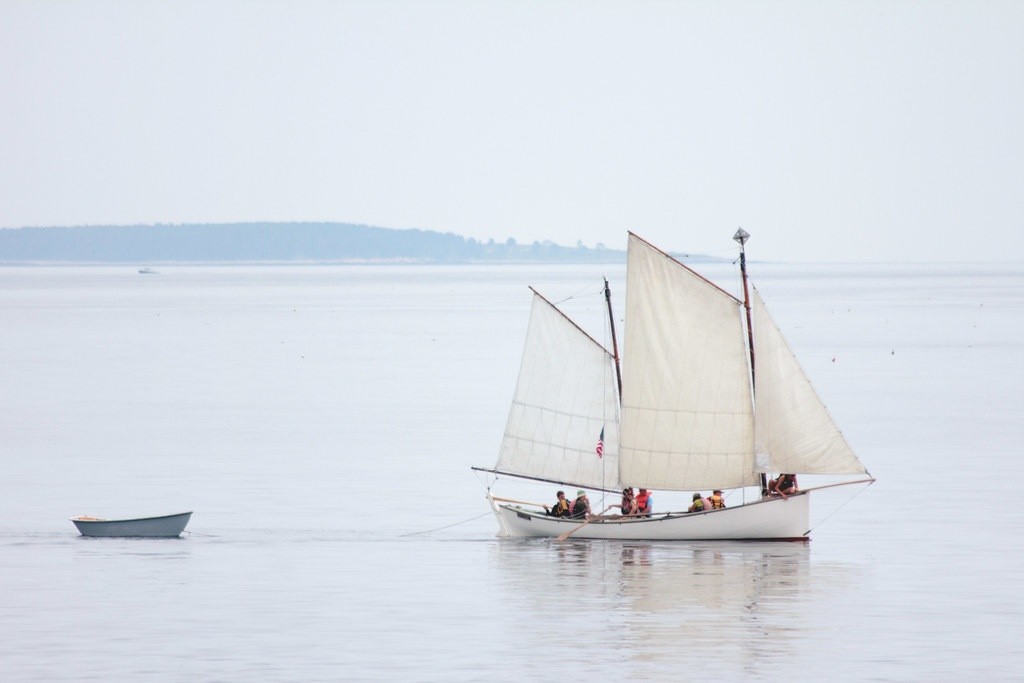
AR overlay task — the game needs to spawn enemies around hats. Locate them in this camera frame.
[713,490,724,493]
[576,489,586,499]
[692,493,700,498]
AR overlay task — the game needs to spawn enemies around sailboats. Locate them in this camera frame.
[464,224,875,543]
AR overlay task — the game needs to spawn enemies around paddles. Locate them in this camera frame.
[557,506,611,540]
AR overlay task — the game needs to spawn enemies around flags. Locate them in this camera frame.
[596,427,604,459]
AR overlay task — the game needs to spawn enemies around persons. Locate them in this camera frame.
[768,474,800,501]
[549,489,571,515]
[687,493,706,514]
[706,490,726,509]
[570,489,591,519]
[635,488,652,519]
[621,488,637,518]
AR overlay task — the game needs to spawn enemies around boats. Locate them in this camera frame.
[71,505,195,542]
